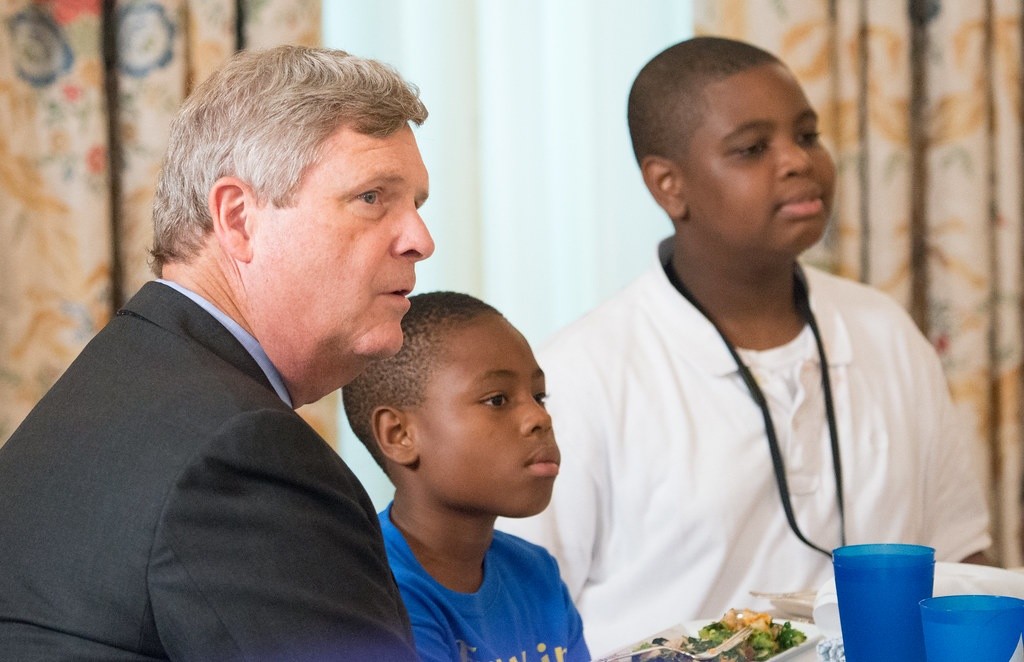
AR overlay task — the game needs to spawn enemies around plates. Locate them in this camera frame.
[588,613,825,662]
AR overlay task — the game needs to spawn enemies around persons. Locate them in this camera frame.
[343,290,592,662]
[0,46,435,662]
[493,39,997,662]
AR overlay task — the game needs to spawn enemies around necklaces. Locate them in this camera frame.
[661,251,847,558]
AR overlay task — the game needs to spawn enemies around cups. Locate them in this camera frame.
[918,594,1024,662]
[832,543,936,662]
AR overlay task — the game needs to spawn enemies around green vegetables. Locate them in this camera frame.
[680,621,807,662]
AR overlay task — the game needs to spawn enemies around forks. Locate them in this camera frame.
[598,626,754,662]
